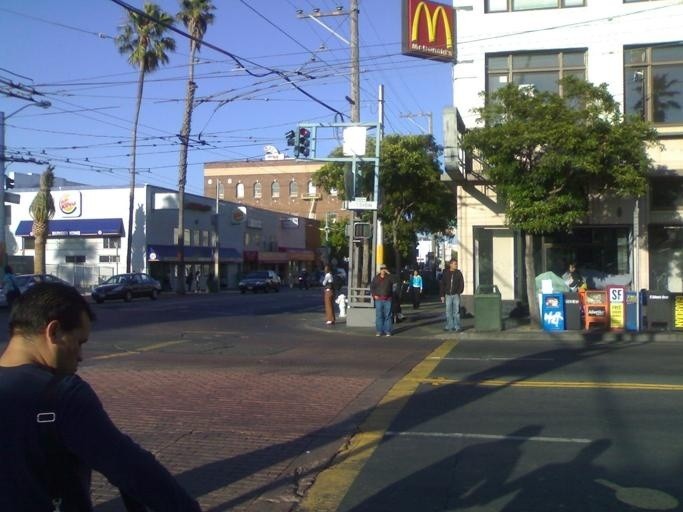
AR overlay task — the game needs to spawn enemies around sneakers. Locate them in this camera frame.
[375,331,393,338]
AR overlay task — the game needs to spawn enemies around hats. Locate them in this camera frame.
[380,263,387,270]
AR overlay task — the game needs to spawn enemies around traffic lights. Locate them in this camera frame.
[285,130,299,158]
[354,223,371,239]
[299,126,311,159]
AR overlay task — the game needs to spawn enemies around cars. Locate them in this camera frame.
[0,274,74,309]
[309,266,346,291]
[91,272,162,304]
[236,268,282,295]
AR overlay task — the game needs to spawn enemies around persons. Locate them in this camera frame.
[439,259,464,331]
[391,282,403,321]
[568,262,584,286]
[321,264,335,325]
[370,263,392,338]
[299,268,310,290]
[0,282,204,512]
[188,271,193,291]
[408,269,423,312]
[195,271,199,288]
[2,266,19,304]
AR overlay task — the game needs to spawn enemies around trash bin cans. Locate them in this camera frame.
[474,284,502,332]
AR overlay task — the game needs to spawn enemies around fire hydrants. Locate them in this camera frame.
[334,293,348,319]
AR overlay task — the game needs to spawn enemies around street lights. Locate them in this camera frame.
[0,101,53,268]
